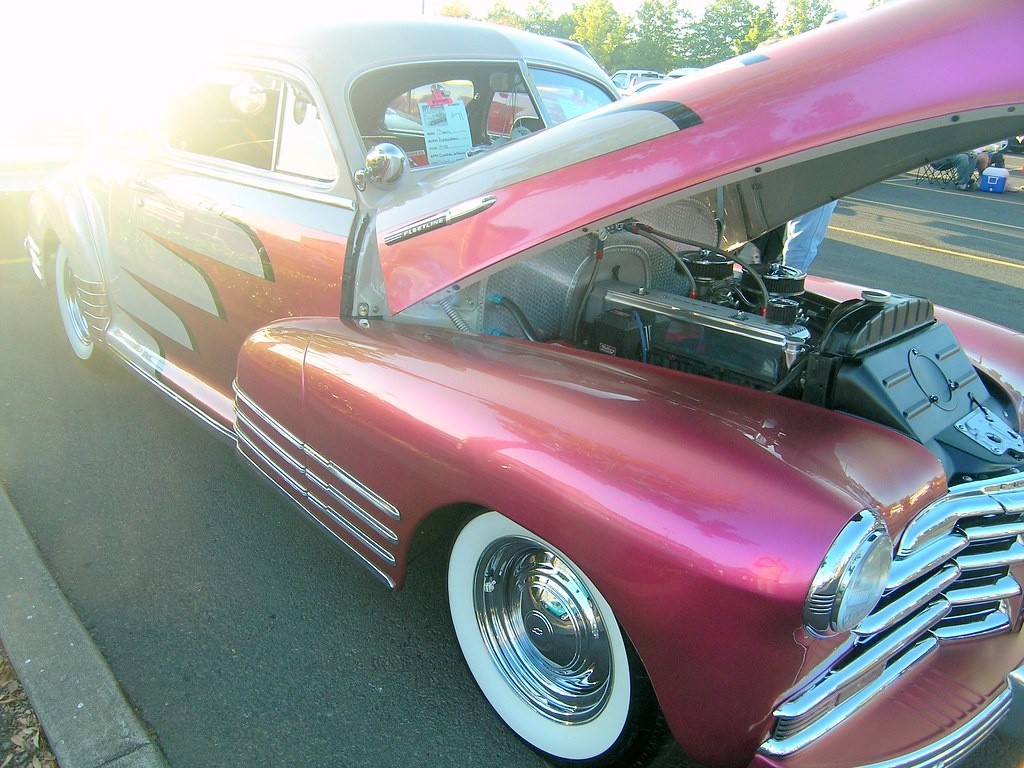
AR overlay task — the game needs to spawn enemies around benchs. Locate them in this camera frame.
[212,135,399,173]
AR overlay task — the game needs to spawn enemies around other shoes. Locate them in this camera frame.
[957,179,975,191]
[1021,186,1023,189]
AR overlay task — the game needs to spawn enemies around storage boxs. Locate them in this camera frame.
[979,167,1009,194]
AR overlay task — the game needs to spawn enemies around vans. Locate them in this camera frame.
[610,70,667,93]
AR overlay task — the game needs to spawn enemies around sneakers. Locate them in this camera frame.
[1005,182,1019,192]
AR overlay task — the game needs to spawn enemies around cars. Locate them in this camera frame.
[991,136,1024,152]
[22,0,1024,768]
[667,68,700,79]
[622,81,664,94]
[626,77,666,90]
[386,80,593,149]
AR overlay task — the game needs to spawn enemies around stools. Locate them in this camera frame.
[915,163,978,190]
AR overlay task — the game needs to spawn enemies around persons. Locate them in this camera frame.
[930,139,1019,192]
[728,39,837,276]
[1016,134,1024,190]
